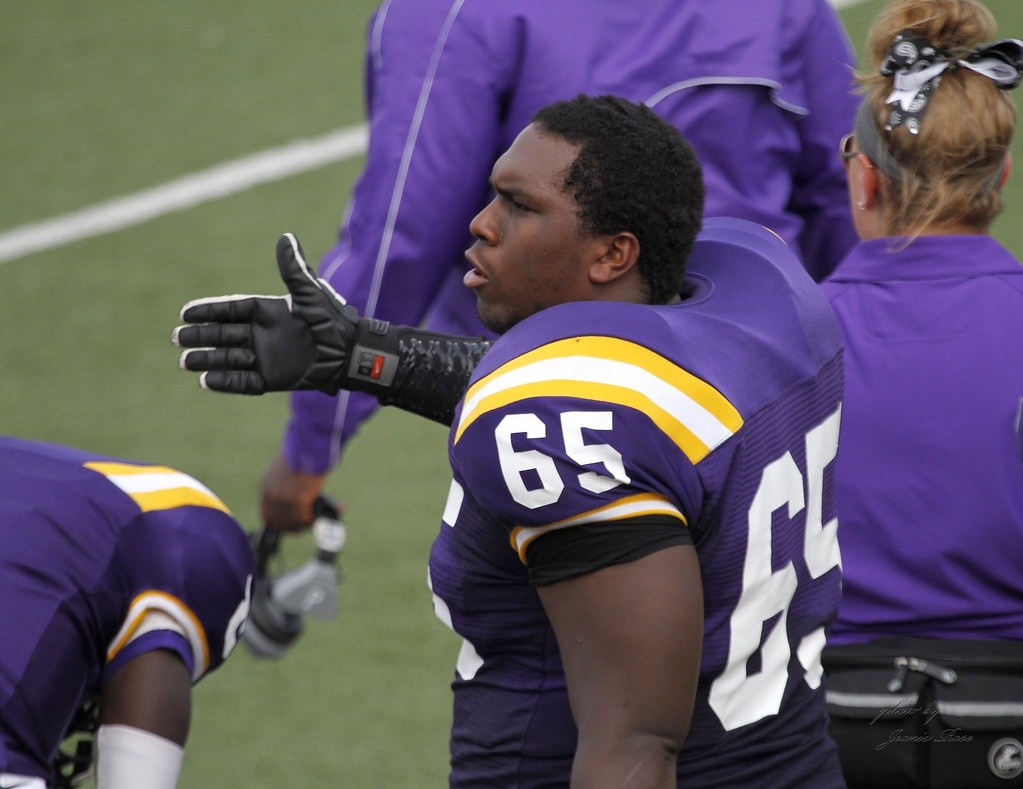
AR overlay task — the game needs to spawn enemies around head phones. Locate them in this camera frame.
[242,493,347,661]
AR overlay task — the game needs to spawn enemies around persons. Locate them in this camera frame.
[169,0,871,789]
[819,0,1023,789]
[0,432,259,789]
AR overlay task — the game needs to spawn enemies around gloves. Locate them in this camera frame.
[169,233,491,427]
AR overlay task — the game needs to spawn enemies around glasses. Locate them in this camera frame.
[840,134,861,166]
